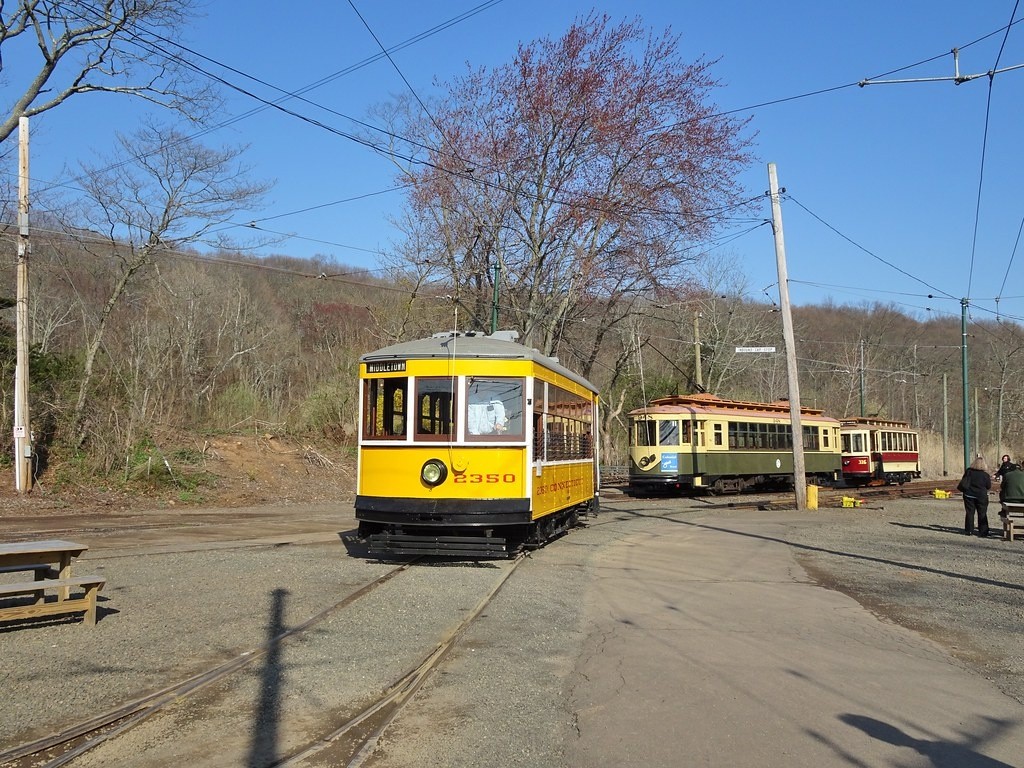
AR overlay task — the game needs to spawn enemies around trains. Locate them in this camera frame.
[622,336,846,498]
[354,306,601,560]
[838,380,921,488]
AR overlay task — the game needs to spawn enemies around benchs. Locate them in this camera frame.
[0,575,105,594]
[0,564,51,573]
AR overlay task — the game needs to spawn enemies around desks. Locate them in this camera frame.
[0,540,105,626]
[1000,502,1024,541]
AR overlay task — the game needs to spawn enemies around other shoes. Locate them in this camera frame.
[965,529,974,535]
[980,532,992,537]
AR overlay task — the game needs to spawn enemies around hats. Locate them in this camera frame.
[1008,464,1021,470]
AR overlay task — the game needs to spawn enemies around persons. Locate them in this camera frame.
[962,457,991,537]
[997,464,1024,515]
[992,455,1014,503]
[467,380,505,435]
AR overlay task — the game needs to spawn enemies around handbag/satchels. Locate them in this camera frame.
[957,469,971,493]
